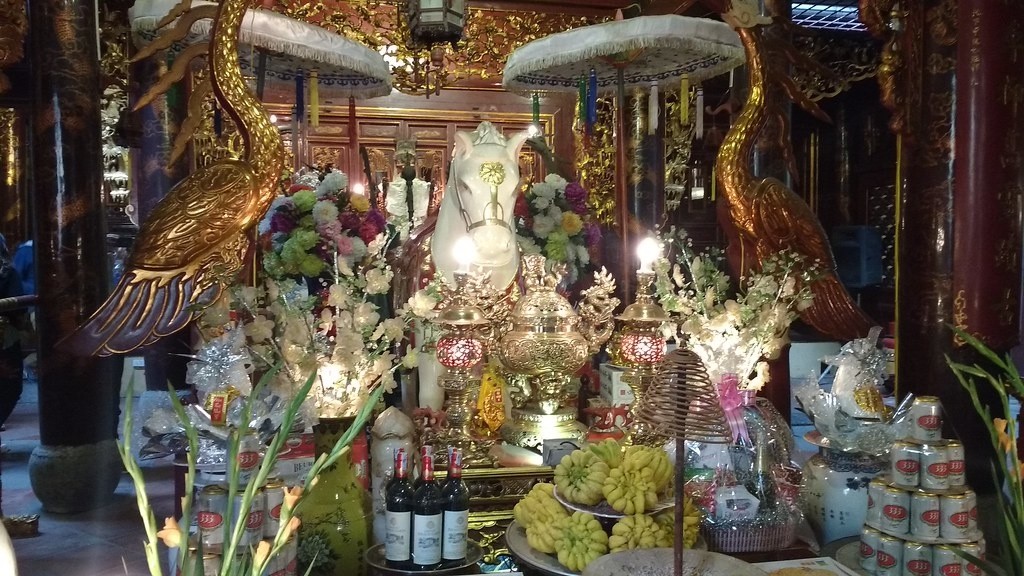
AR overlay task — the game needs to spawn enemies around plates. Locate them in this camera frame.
[506,519,708,576]
[364,539,484,573]
[553,484,674,518]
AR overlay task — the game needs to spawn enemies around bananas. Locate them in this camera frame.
[513,482,702,570]
[553,440,673,512]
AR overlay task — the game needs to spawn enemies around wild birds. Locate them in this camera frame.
[57,0,284,357]
[715,21,880,343]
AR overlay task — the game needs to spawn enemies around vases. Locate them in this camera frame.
[292,416,372,576]
[311,305,337,338]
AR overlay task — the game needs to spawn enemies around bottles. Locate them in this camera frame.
[413,445,443,572]
[441,448,469,570]
[386,448,413,569]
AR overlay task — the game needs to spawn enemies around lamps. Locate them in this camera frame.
[612,237,675,449]
[426,235,499,471]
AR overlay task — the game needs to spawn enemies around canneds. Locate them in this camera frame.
[858,395,987,576]
[173,430,296,576]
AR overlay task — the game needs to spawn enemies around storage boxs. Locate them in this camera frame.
[586,397,631,432]
[599,363,636,408]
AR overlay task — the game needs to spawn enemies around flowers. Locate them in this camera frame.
[513,173,601,299]
[177,260,443,418]
[654,222,844,392]
[945,321,1024,575]
[112,370,384,576]
[257,169,393,305]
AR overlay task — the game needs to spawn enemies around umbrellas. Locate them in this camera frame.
[503,9,745,310]
[128,1,392,105]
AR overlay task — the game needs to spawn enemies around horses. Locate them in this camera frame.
[399,118,542,410]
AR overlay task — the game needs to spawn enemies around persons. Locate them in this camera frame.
[108,247,127,287]
[0,233,44,537]
